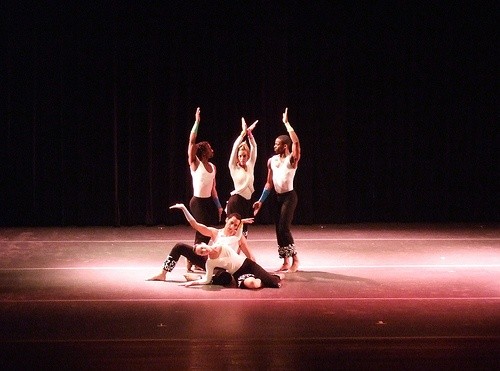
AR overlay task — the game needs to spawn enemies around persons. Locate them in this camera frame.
[224,117,257,240]
[185,106,223,273]
[144,204,285,288]
[253,107,300,272]
